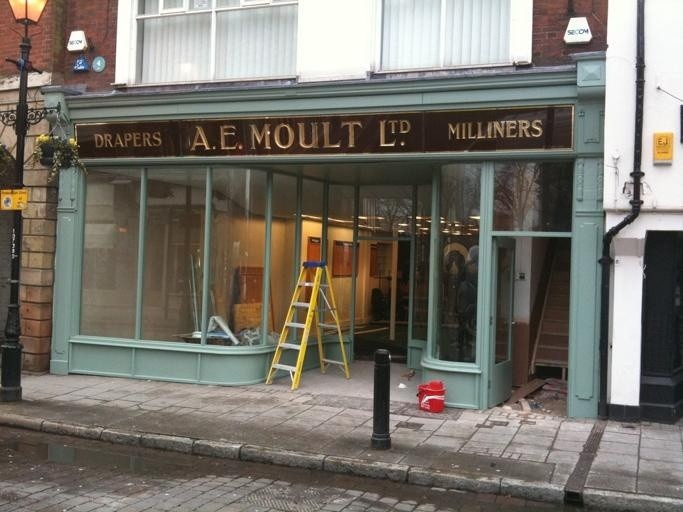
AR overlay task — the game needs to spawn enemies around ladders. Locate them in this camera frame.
[265,262,350,390]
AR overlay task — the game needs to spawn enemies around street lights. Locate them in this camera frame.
[0,0,48,404]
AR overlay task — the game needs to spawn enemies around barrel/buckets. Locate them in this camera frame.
[416,380,446,413]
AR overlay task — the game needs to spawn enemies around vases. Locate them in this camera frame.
[39,144,73,169]
[0,161,20,184]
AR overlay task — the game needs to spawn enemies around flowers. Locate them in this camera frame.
[0,142,19,177]
[31,133,89,182]
